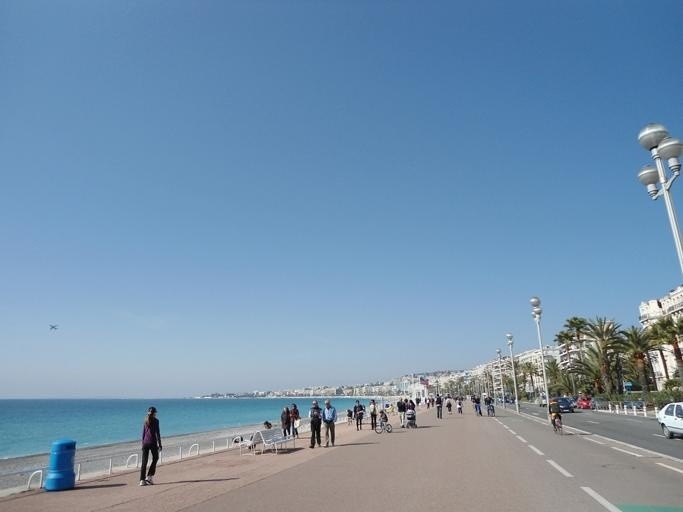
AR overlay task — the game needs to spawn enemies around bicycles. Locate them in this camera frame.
[547,410,562,435]
[374,418,392,433]
[486,403,495,417]
[475,403,480,416]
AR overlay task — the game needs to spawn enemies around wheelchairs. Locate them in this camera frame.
[404,412,417,429]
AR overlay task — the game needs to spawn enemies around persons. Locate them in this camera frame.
[347,391,496,428]
[309,400,323,448]
[290,404,300,438]
[139,407,161,485]
[281,408,291,437]
[322,400,338,448]
[547,398,563,431]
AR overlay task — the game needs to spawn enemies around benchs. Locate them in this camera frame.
[240,428,297,456]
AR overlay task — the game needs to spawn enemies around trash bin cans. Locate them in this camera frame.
[385,404,389,408]
[43,438,77,492]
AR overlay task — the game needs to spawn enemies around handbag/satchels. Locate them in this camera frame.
[294,419,301,428]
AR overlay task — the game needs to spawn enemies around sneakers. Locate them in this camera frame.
[139,476,152,486]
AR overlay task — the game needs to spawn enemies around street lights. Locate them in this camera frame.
[636,124,683,273]
[495,348,505,407]
[504,332,521,414]
[529,297,550,416]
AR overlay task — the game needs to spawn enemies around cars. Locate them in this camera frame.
[656,401,683,439]
[534,393,644,413]
